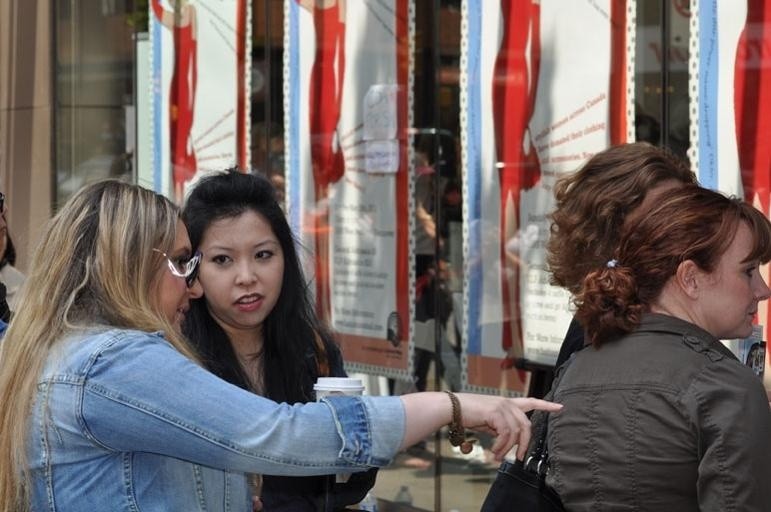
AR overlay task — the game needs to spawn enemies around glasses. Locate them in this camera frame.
[152,247,202,289]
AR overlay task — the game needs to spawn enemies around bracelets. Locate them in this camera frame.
[445,391,472,453]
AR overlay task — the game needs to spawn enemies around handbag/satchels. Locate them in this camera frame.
[480,351,578,512]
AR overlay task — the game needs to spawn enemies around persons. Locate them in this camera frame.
[505,180,566,402]
[179,167,382,509]
[0,178,568,508]
[544,141,743,379]
[480,185,771,509]
[1,190,27,336]
[406,149,456,458]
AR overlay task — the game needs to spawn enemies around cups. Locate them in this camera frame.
[313,377,363,402]
